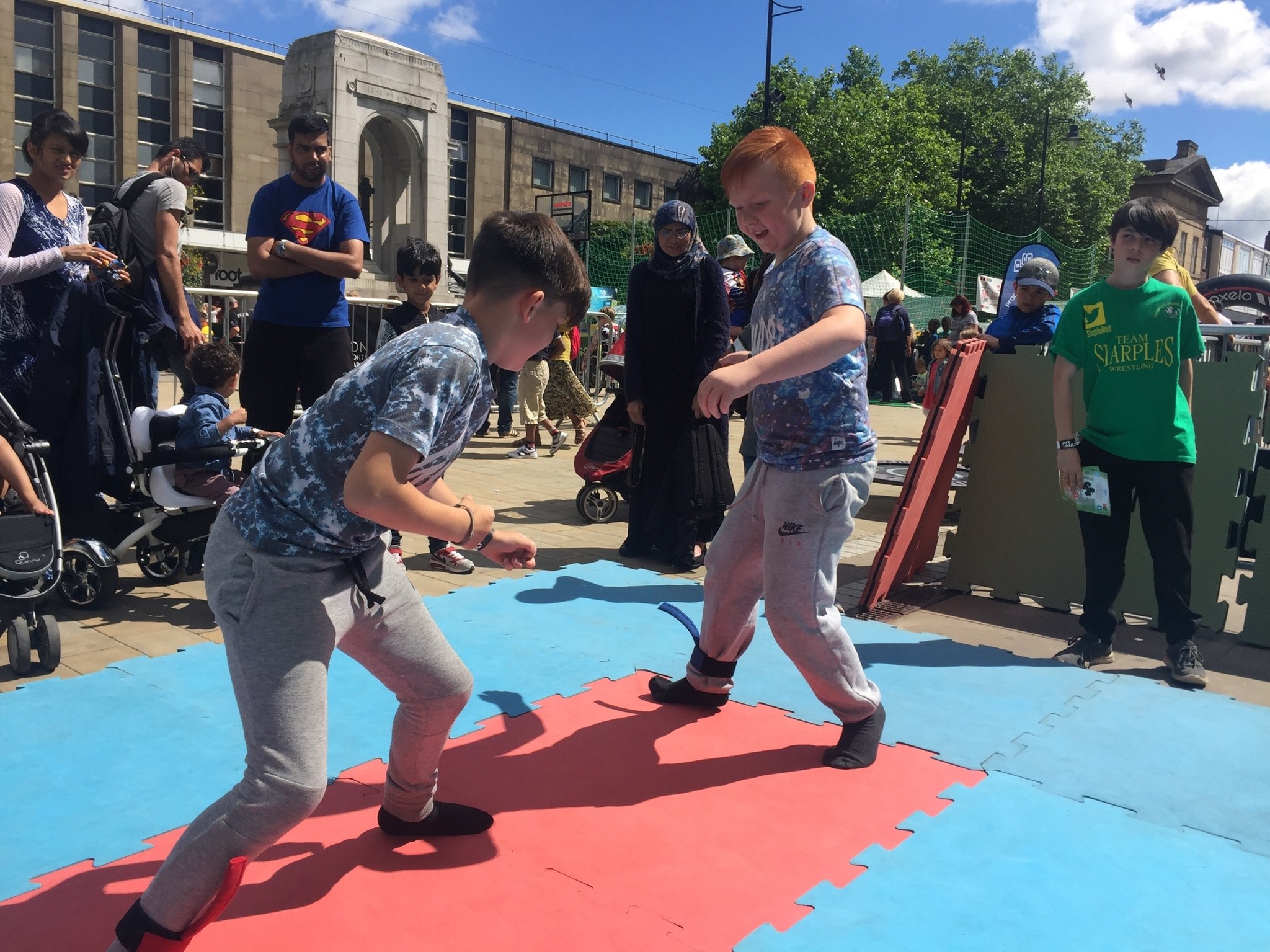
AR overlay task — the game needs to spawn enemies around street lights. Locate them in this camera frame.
[954,125,1011,268]
[1032,105,1087,243]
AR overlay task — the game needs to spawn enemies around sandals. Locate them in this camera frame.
[574,418,587,444]
[513,437,542,446]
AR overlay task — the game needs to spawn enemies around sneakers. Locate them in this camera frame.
[1165,639,1210,683]
[429,544,475,574]
[389,545,407,575]
[549,431,569,455]
[507,445,538,459]
[1055,634,1114,667]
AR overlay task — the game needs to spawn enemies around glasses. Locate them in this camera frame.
[181,152,201,185]
[659,228,690,239]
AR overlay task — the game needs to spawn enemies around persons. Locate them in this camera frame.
[237,110,371,474]
[0,108,283,592]
[1149,244,1234,352]
[618,200,736,567]
[103,210,592,952]
[1047,197,1208,687]
[374,241,476,577]
[478,300,624,460]
[648,127,1064,770]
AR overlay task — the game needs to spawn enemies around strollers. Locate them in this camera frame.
[0,391,65,676]
[573,318,640,524]
[53,287,286,611]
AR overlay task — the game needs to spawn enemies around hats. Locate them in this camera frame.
[1015,257,1060,298]
[716,234,755,260]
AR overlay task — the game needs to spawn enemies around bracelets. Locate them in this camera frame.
[476,529,495,551]
[1056,439,1077,449]
[450,505,475,547]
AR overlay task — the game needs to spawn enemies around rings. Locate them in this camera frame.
[91,249,95,255]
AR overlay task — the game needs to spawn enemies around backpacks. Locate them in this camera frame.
[88,172,169,296]
[673,423,736,520]
[877,305,905,339]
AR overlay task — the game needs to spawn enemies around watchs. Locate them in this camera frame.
[251,427,262,439]
[276,239,287,257]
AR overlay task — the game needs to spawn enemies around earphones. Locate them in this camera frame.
[172,156,175,159]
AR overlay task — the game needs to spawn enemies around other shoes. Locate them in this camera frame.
[679,543,706,568]
[905,400,916,404]
[619,536,639,556]
[476,426,492,437]
[880,400,891,404]
[498,428,520,438]
